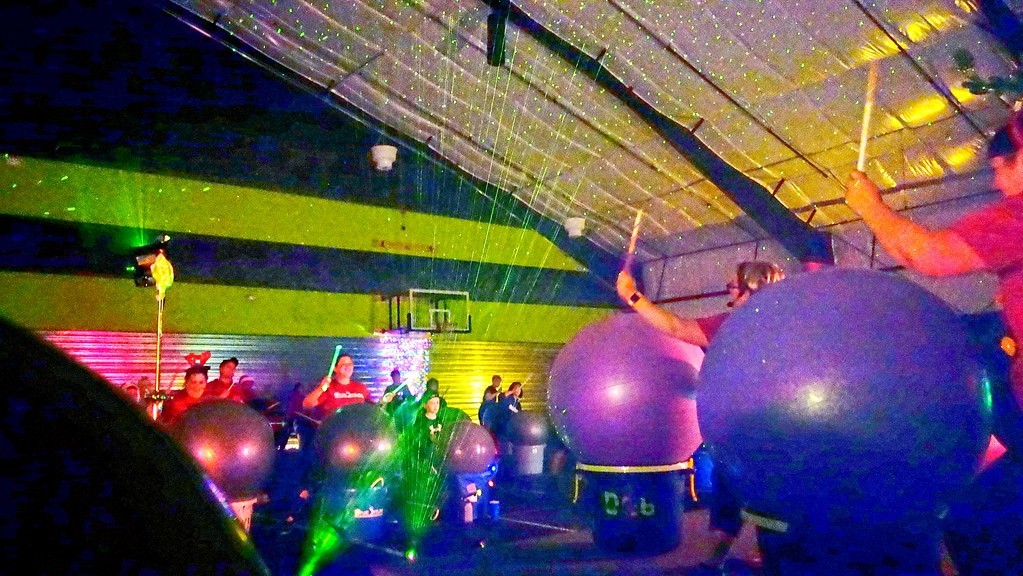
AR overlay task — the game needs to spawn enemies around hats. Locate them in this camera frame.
[220,357,239,368]
[424,392,438,403]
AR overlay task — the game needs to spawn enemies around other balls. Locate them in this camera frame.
[695,270,997,531]
[322,404,398,473]
[547,311,703,466]
[506,409,550,446]
[171,398,276,501]
[438,422,494,473]
[0,313,262,576]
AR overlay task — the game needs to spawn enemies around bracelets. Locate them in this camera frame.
[627,290,643,308]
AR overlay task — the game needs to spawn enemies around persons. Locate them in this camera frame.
[116,353,525,524]
[842,105,1023,576]
[615,259,785,570]
